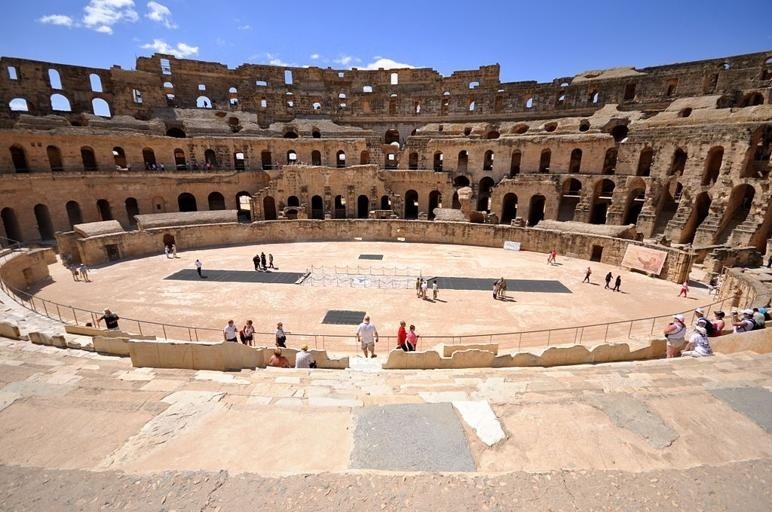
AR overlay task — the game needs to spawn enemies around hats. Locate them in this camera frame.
[672,313,685,323]
[741,309,754,315]
[694,307,705,315]
[713,310,725,318]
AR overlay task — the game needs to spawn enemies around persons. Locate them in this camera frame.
[416,278,439,300]
[187,160,214,170]
[172,243,176,257]
[677,279,689,298]
[242,320,256,346]
[70,264,81,281]
[268,348,292,368]
[407,325,419,351]
[665,308,767,358]
[96,308,119,330]
[276,322,286,349]
[224,320,238,342]
[357,315,378,358]
[165,245,170,258]
[126,162,132,170]
[550,247,556,264]
[612,275,621,291]
[194,259,202,276]
[582,266,592,283]
[253,252,273,270]
[604,272,613,289]
[144,162,164,171]
[79,264,91,282]
[395,321,408,352]
[547,252,553,266]
[85,322,92,326]
[688,243,695,253]
[295,345,317,368]
[493,278,506,300]
